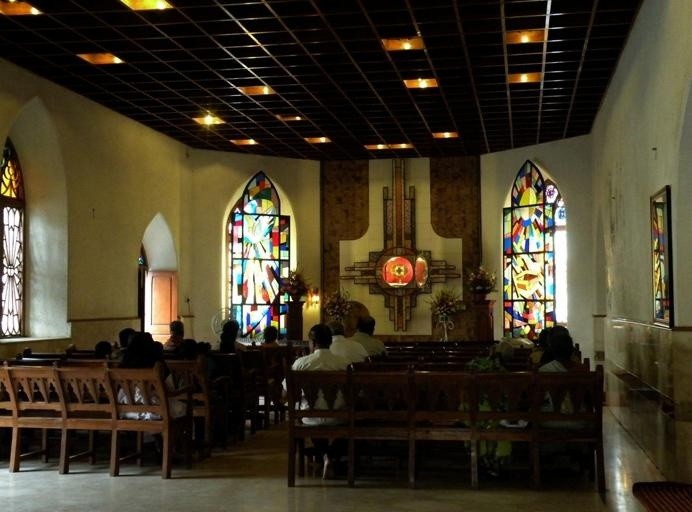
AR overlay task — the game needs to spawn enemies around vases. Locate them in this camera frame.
[284,301,305,342]
[436,315,454,341]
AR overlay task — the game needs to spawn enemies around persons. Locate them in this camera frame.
[281,316,386,479]
[458,327,589,476]
[261,325,279,348]
[212,319,247,352]
[163,321,211,394]
[93,326,178,422]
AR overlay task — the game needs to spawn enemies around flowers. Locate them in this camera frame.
[278,261,315,301]
[320,285,353,321]
[423,261,499,316]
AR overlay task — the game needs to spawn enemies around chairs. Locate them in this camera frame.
[2,359,194,478]
[284,361,606,490]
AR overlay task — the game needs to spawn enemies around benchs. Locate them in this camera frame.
[284,339,607,457]
[1,338,310,450]
